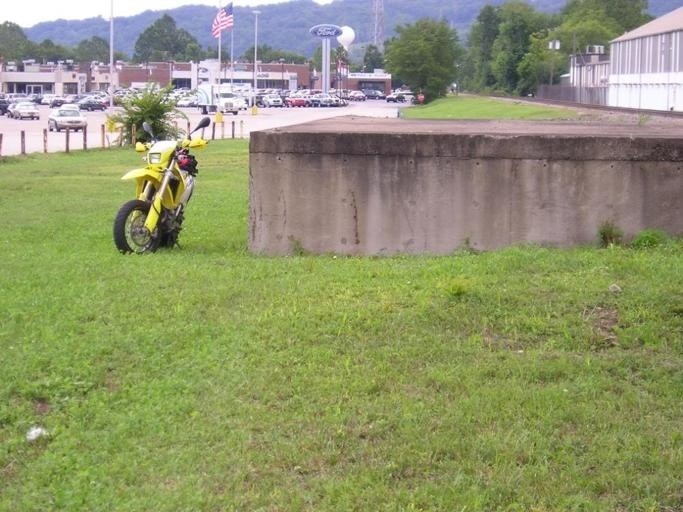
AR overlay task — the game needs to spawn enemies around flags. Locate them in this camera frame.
[211,3,234,38]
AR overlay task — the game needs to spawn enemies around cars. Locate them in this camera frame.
[48,110,87,132]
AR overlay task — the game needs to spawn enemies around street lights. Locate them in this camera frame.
[252,10,261,89]
[337,58,343,106]
[168,61,173,84]
[192,60,200,89]
[279,58,285,91]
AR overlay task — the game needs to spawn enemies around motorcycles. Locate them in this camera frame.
[114,117,210,255]
